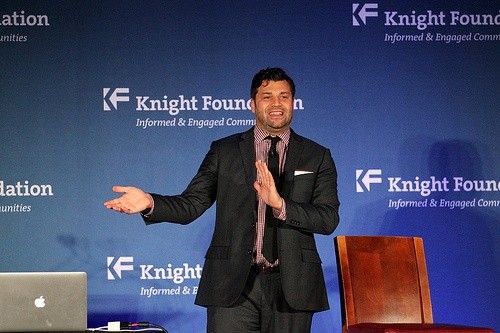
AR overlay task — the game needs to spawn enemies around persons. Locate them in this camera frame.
[103,66,341,333]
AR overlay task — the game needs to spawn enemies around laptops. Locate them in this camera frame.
[0,271,88,330]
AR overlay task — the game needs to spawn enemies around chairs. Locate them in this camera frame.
[335,233,496,333]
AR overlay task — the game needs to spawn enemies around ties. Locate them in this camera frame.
[263,135,281,264]
